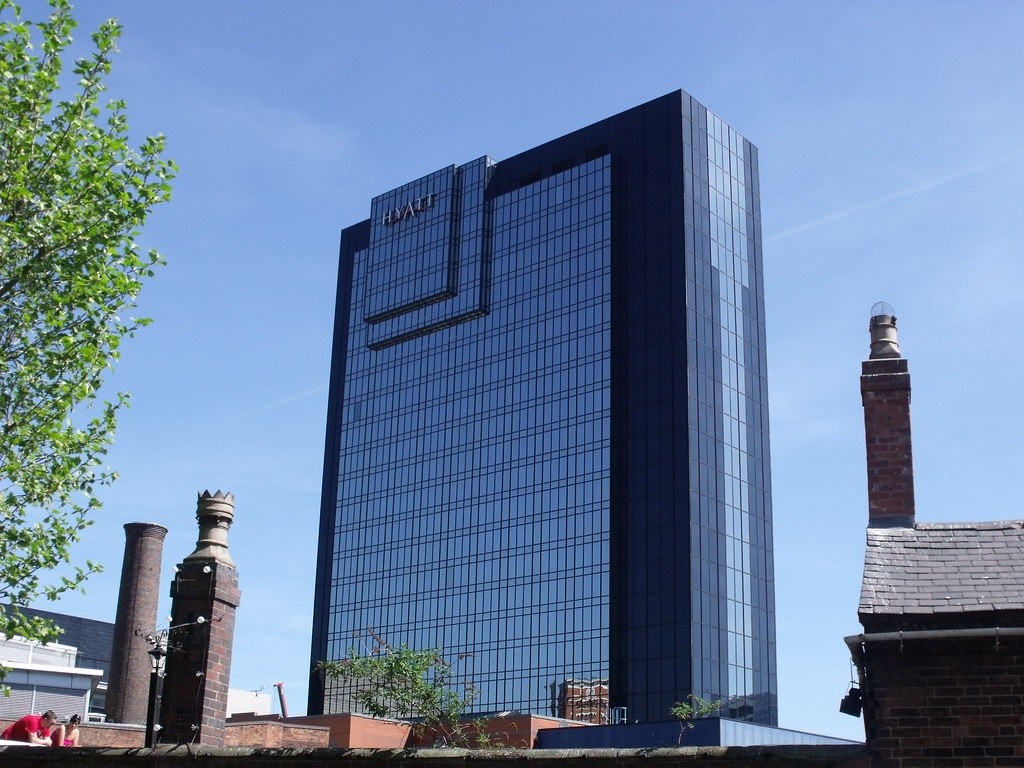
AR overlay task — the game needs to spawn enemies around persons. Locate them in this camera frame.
[50,714,81,746]
[2,710,57,746]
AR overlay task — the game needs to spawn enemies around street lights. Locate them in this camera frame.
[145,642,168,749]
